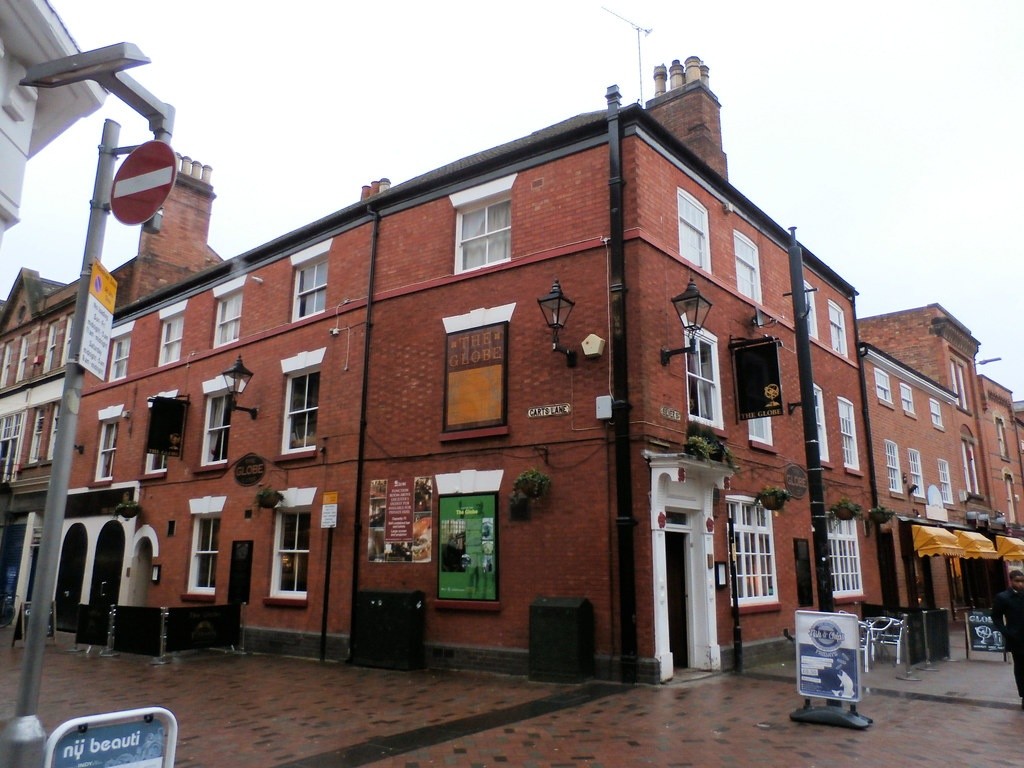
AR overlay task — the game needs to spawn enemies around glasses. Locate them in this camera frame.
[1013,578,1024,582]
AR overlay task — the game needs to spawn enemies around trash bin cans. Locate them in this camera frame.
[527,594,595,684]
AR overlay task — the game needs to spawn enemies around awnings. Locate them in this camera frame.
[996,535,1024,559]
[912,524,965,557]
[954,530,998,559]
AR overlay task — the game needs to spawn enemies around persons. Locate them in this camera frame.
[992,570,1024,709]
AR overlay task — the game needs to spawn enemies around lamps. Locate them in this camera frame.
[222,354,258,419]
[660,276,714,366]
[537,278,577,369]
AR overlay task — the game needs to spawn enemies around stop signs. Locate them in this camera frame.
[110,139,177,227]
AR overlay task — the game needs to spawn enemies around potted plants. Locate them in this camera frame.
[255,489,284,508]
[829,497,861,521]
[752,484,792,511]
[114,500,143,518]
[685,422,740,475]
[868,505,895,524]
[513,468,551,499]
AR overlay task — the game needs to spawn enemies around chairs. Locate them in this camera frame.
[837,611,904,673]
